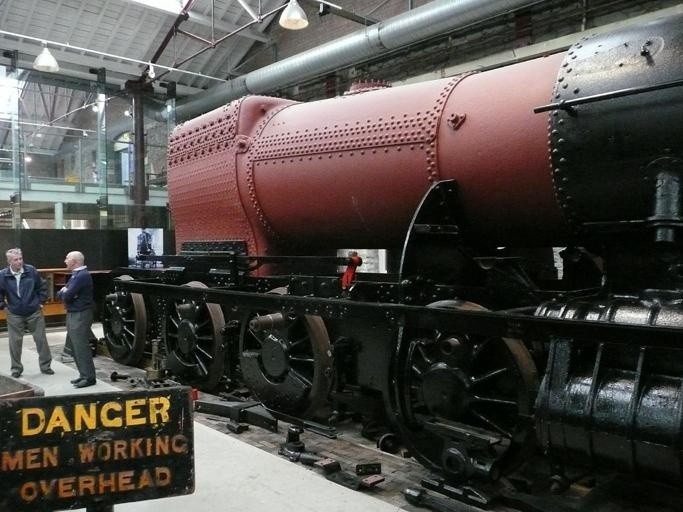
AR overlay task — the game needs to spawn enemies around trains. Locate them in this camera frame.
[99,0,681,508]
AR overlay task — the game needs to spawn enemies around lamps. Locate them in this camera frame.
[32,46,59,74]
[278,0,309,32]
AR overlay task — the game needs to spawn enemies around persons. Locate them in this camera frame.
[58,251,96,388]
[136,228,156,264]
[0,248,55,378]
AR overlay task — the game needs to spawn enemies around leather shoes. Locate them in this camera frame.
[70,377,83,384]
[12,372,21,378]
[40,368,54,374]
[73,378,96,388]
